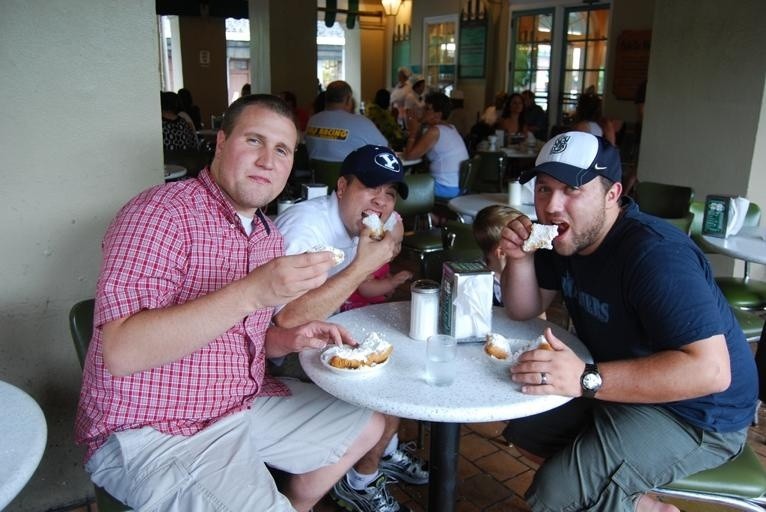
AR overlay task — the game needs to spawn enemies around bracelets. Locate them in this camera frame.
[407,133,415,142]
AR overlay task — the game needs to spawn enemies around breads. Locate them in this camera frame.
[309,244,347,267]
[523,335,554,356]
[366,207,385,240]
[486,330,512,361]
[330,332,394,372]
[521,223,560,253]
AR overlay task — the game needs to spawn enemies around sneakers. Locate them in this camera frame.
[329,467,413,512]
[378,450,430,484]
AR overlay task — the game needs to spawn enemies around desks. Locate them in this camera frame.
[704,234,766,278]
[449,193,508,219]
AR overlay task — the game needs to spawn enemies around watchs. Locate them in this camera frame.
[581,361,601,400]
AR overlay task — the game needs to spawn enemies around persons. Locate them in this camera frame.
[340,254,413,310]
[404,92,473,198]
[497,133,759,512]
[311,91,328,112]
[390,67,409,116]
[496,93,528,139]
[519,90,547,139]
[367,90,403,141]
[406,75,427,122]
[624,81,646,198]
[277,91,307,132]
[302,82,392,161]
[75,97,385,512]
[160,88,203,175]
[466,107,499,158]
[473,204,534,307]
[571,116,622,146]
[273,143,433,512]
[446,88,474,137]
[241,84,251,95]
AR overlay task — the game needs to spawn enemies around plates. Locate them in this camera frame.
[317,342,394,380]
[481,334,552,374]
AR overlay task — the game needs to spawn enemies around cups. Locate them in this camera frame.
[423,333,460,387]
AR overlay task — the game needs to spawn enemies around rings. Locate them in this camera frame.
[540,372,546,383]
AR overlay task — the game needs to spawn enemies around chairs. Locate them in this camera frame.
[313,160,344,195]
[644,441,766,512]
[718,280,766,342]
[689,202,761,243]
[395,176,446,279]
[421,225,483,282]
[460,153,507,194]
[70,299,131,512]
[632,183,695,239]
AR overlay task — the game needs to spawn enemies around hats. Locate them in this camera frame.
[411,75,424,88]
[519,132,621,189]
[400,67,412,78]
[340,145,409,200]
